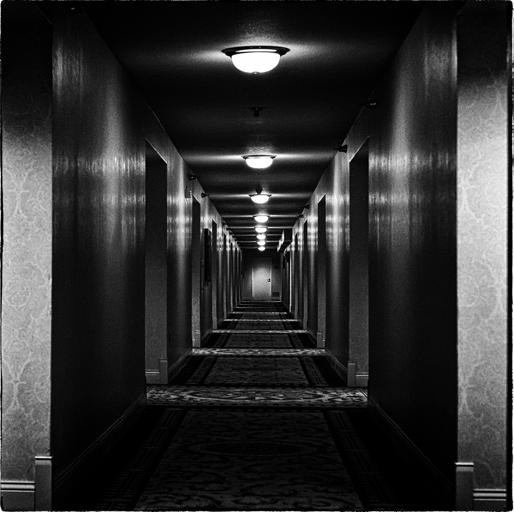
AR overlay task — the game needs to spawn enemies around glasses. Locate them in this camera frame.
[246,157,274,170]
[251,194,269,204]
[228,49,280,75]
[254,216,268,232]
